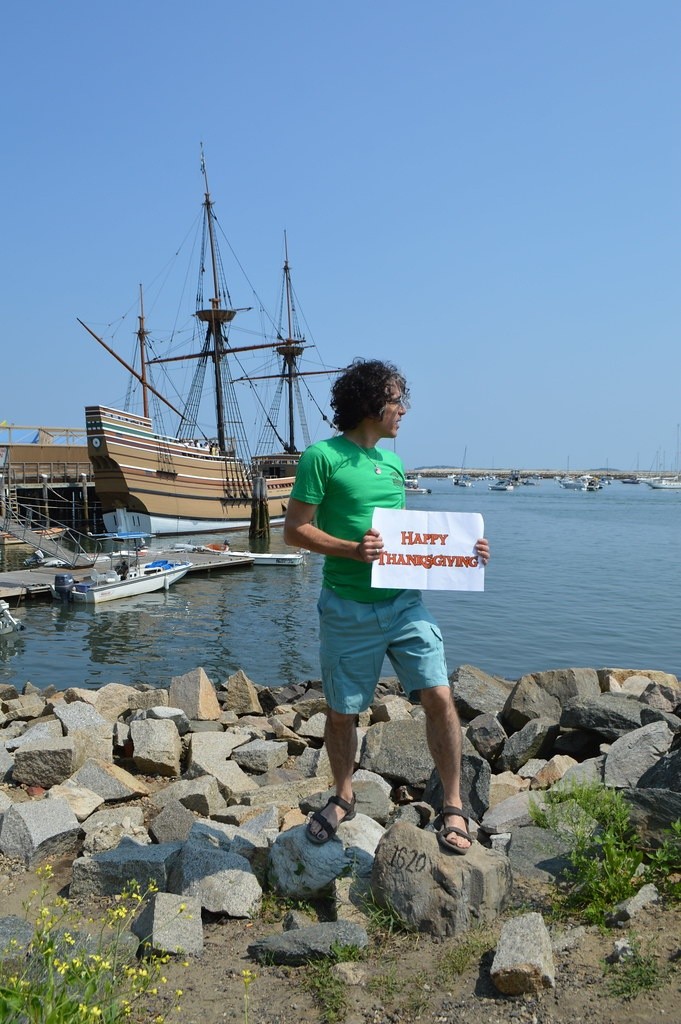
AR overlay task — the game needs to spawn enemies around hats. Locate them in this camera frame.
[122,561,126,565]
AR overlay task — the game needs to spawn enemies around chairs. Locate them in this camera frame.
[106,570,120,583]
[89,567,105,586]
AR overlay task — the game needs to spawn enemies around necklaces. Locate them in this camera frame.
[351,438,382,475]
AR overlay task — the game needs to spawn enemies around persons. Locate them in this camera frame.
[223,540,230,552]
[173,439,220,461]
[283,358,490,854]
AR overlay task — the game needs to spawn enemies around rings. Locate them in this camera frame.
[376,549,380,555]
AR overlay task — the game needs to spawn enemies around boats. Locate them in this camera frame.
[197,546,305,565]
[70,558,193,605]
[35,542,256,569]
[487,479,515,492]
[554,471,615,494]
[445,471,543,496]
[621,475,640,484]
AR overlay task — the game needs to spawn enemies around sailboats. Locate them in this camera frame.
[635,423,681,490]
[80,139,351,535]
[453,446,473,487]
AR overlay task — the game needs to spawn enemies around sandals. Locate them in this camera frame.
[440,801,472,855]
[306,792,358,843]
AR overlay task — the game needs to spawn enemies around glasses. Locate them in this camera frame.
[387,396,407,407]
